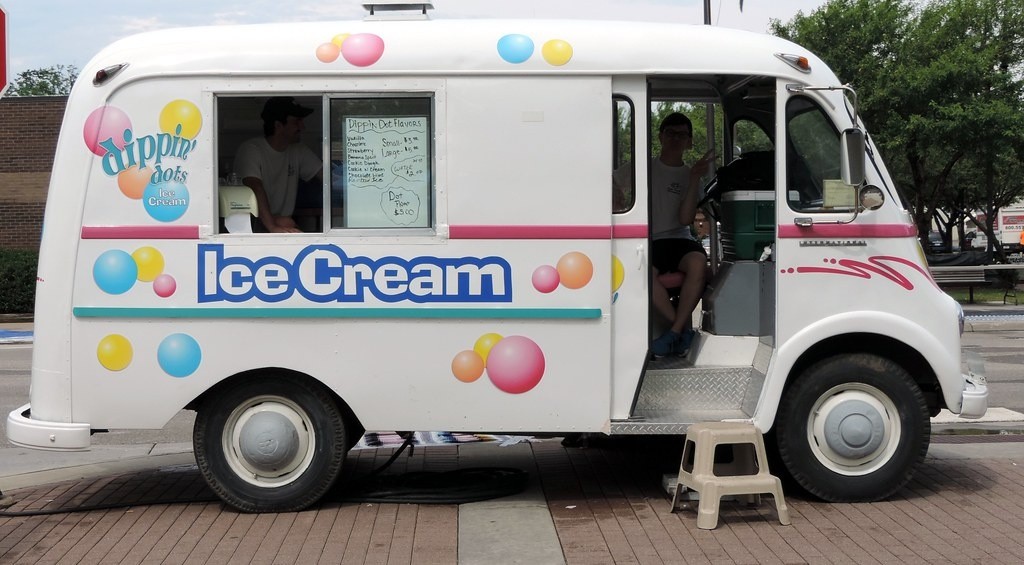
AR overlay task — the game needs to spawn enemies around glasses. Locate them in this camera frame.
[663,127,691,140]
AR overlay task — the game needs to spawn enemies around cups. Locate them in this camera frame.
[224,170,242,186]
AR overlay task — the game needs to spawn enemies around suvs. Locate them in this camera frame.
[927,230,946,252]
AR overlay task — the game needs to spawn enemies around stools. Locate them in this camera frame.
[669,422,791,527]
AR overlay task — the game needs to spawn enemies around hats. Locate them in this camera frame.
[659,113,694,151]
[263,96,315,118]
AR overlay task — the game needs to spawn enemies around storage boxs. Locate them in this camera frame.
[720,188,799,261]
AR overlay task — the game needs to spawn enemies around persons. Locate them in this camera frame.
[230,97,341,233]
[613,112,721,360]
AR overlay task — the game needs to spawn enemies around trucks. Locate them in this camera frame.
[964,195,1024,253]
[7,2,989,514]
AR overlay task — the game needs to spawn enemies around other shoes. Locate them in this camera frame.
[676,329,700,356]
[648,332,676,360]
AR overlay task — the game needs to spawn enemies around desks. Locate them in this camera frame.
[982,263,1024,306]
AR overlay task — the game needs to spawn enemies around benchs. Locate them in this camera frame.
[929,249,993,304]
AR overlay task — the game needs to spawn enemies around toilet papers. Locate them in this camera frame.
[218,185,258,233]
